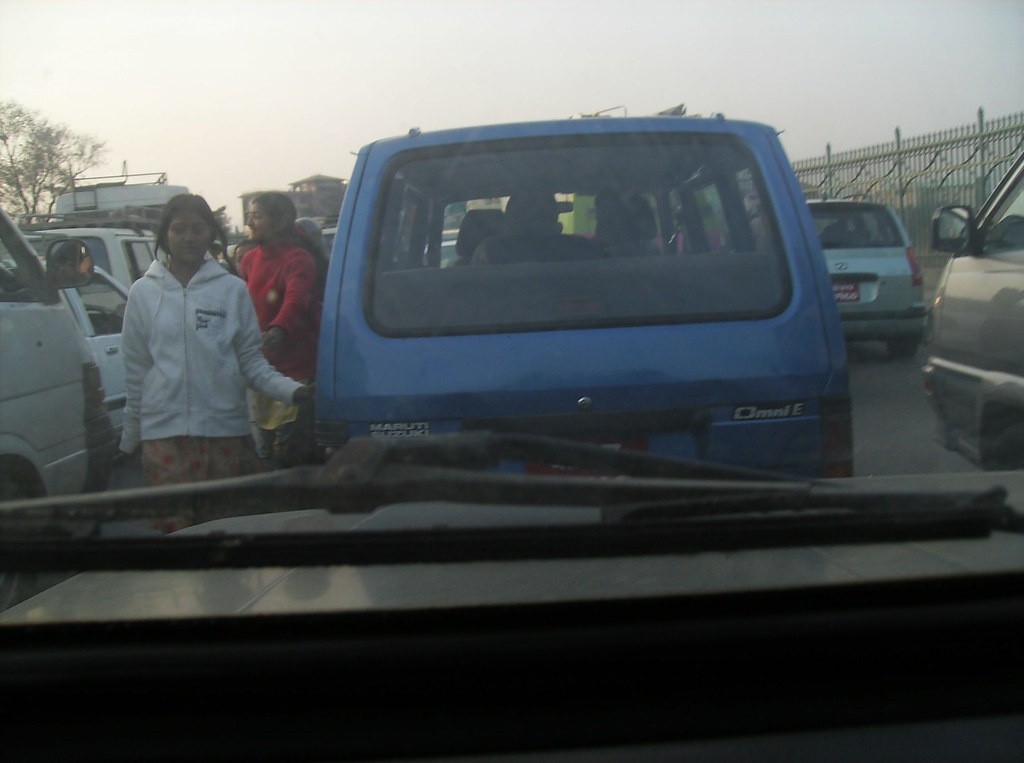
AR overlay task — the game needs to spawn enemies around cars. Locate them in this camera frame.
[0,207,118,505]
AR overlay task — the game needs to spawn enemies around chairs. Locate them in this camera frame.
[823,224,870,248]
[368,196,769,329]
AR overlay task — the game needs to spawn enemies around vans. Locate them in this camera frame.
[316,118,854,481]
[920,147,1024,498]
[18,206,218,454]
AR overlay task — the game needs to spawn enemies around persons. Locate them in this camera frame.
[50,243,83,285]
[118,191,329,530]
[473,184,658,268]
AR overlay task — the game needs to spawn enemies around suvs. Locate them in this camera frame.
[804,193,926,361]
